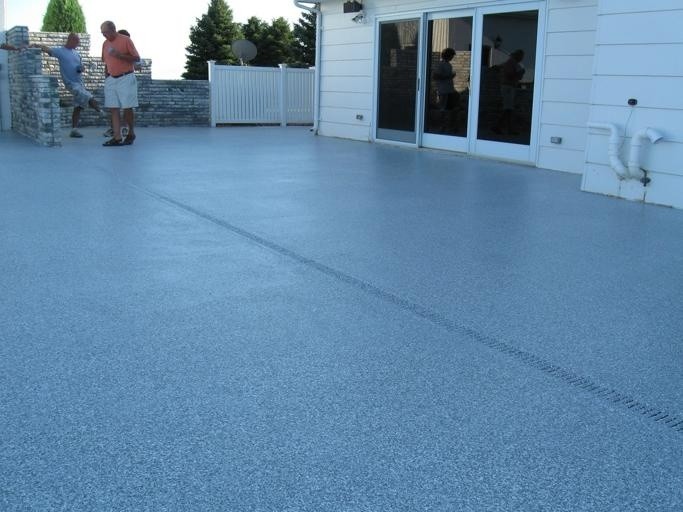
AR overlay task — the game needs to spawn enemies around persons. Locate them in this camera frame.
[432,46,459,110]
[98,20,142,147]
[98,29,136,137]
[32,33,107,139]
[492,43,525,137]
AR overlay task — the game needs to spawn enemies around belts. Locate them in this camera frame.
[111,70,133,78]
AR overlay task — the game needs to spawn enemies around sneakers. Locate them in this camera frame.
[103,127,135,146]
[69,129,83,138]
[99,110,110,119]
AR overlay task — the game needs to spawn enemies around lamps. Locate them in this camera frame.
[343,0,363,13]
